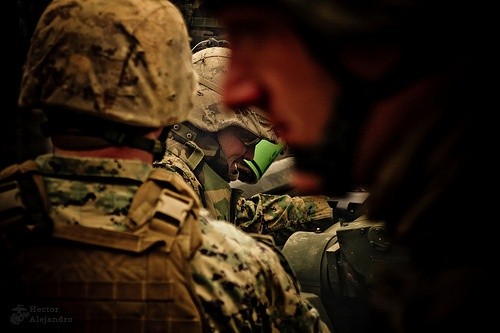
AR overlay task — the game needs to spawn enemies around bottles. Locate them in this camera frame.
[237,138,287,184]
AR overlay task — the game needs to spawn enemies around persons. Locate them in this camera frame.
[1,0,499,333]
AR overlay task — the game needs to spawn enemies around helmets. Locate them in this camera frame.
[183,45,280,145]
[195,0,498,96]
[18,0,200,126]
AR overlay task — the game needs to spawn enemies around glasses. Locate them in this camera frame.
[230,128,261,147]
